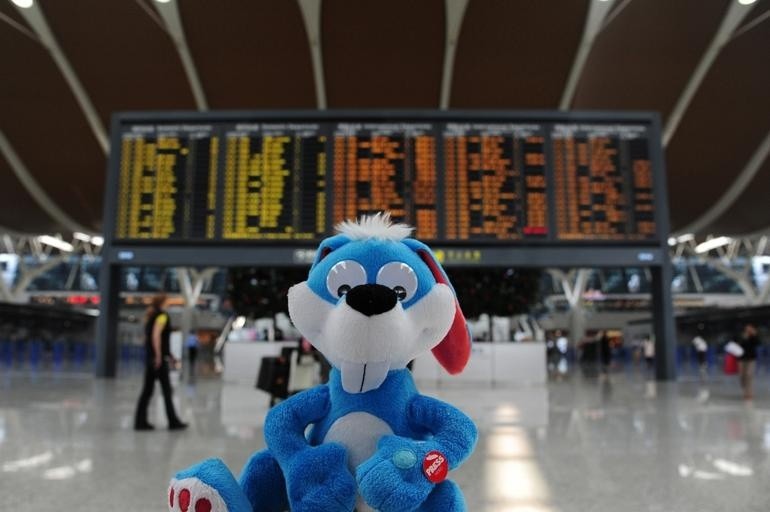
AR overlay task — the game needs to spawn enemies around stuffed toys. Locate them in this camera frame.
[166,212,479,512]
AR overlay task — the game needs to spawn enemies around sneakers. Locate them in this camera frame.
[168,420,189,431]
[134,421,155,431]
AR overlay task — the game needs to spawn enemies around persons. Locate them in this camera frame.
[737,319,763,403]
[133,296,190,432]
[602,329,711,369]
